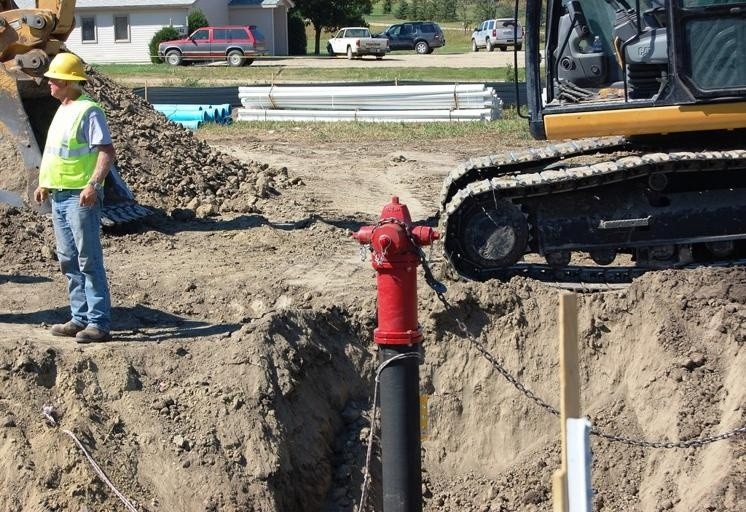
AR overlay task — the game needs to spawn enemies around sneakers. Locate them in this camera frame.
[51,320,84,336]
[75,325,110,344]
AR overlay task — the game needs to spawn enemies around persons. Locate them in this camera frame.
[28,52,113,344]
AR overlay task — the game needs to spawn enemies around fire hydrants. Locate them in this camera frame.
[353,197,440,347]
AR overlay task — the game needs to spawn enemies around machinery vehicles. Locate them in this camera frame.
[1,0,746,296]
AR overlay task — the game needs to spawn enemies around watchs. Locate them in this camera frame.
[86,179,103,193]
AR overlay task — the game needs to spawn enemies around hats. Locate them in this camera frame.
[43,52,91,81]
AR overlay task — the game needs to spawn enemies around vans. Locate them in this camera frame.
[470,18,524,52]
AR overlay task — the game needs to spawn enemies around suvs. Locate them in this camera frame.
[157,25,268,67]
[380,21,446,55]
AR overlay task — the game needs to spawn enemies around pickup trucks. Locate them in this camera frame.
[326,26,391,60]
